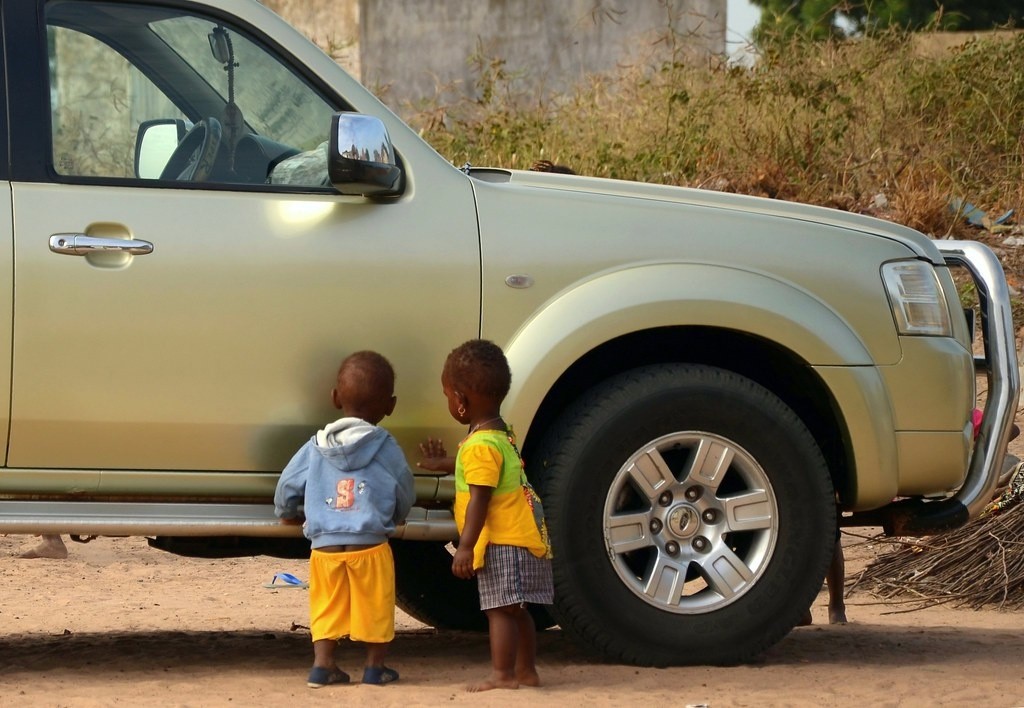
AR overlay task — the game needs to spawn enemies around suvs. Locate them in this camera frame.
[0,0,1024,670]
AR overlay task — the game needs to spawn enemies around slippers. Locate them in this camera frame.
[16,548,39,558]
[261,572,309,590]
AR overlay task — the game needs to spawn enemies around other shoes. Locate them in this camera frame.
[363,665,399,685]
[307,662,350,688]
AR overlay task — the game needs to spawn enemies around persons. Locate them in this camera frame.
[416,339,552,693]
[273,350,415,688]
[19,534,68,559]
[973,409,1021,443]
